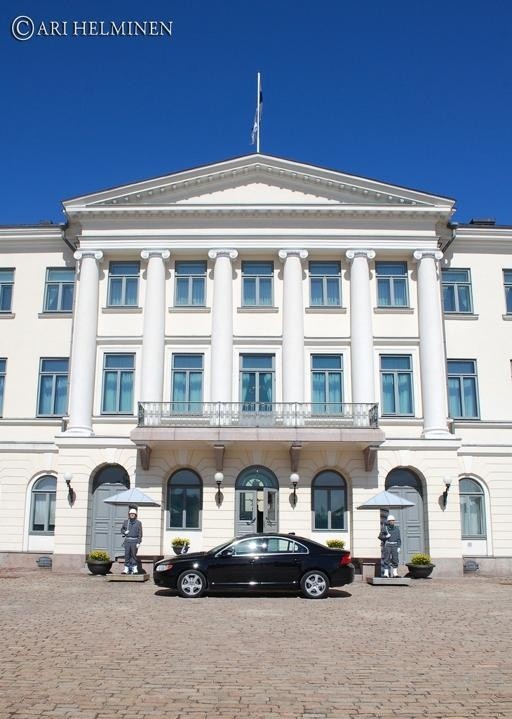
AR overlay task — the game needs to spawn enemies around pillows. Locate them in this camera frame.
[387,515,396,522]
[128,508,136,514]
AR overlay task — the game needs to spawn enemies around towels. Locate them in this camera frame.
[153,556,164,571]
[362,562,376,583]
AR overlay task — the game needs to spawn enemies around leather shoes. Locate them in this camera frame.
[135,544,140,549]
[386,534,391,539]
[397,548,401,553]
[125,531,129,534]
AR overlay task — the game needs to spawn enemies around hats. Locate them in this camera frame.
[152,532,356,600]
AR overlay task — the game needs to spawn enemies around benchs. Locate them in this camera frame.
[249,75,264,146]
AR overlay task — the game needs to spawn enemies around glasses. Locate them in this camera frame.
[214,471,224,503]
[63,473,74,502]
[442,474,452,505]
[290,472,300,504]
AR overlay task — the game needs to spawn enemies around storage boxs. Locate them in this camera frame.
[115,555,164,567]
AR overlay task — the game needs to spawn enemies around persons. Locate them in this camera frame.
[376,515,402,577]
[120,505,143,575]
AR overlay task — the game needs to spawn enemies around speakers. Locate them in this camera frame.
[133,565,138,574]
[383,569,389,578]
[120,566,130,574]
[393,568,401,578]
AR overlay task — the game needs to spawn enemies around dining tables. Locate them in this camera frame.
[86,550,116,575]
[171,537,191,554]
[405,552,436,578]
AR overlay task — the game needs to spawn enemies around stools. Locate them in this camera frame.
[387,542,398,544]
[126,537,137,539]
